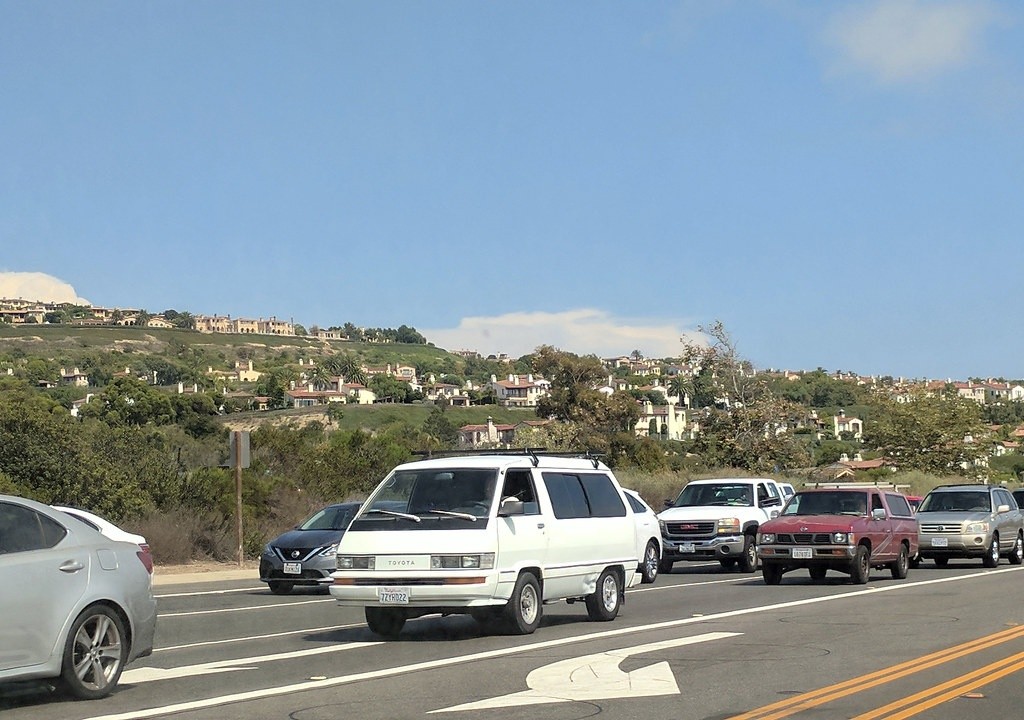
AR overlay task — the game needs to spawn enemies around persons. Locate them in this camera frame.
[854,496,873,517]
[472,476,519,512]
[969,494,990,509]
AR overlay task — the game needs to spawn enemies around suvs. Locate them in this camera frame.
[914,483,1024,568]
[776,482,798,504]
[755,482,920,586]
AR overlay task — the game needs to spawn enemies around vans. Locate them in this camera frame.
[1010,488,1024,517]
[328,446,644,636]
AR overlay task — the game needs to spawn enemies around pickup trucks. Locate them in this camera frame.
[655,478,800,574]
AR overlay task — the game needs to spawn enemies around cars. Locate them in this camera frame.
[906,496,923,509]
[48,505,147,545]
[622,487,662,585]
[259,500,409,594]
[0,494,158,701]
